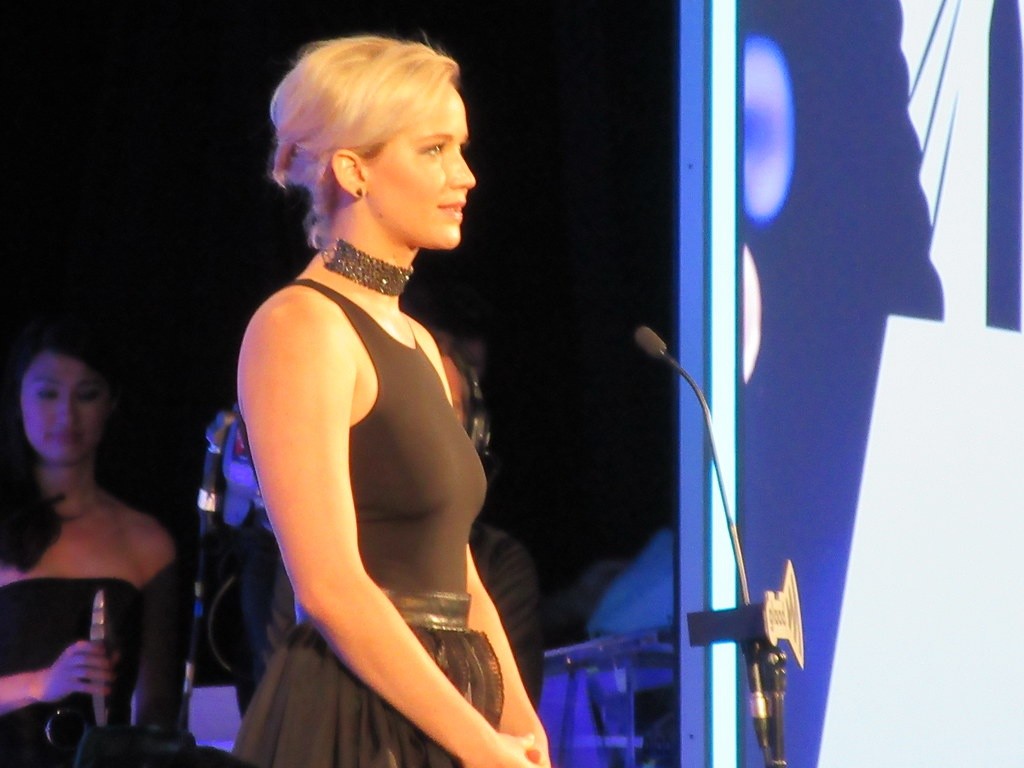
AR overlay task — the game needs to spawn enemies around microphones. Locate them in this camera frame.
[634,324,778,747]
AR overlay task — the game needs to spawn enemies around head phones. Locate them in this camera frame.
[443,347,491,461]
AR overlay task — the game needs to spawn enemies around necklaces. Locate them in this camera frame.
[320,240,413,295]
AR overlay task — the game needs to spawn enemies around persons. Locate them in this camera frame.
[0,313,180,768]
[266,328,544,710]
[230,36,552,768]
